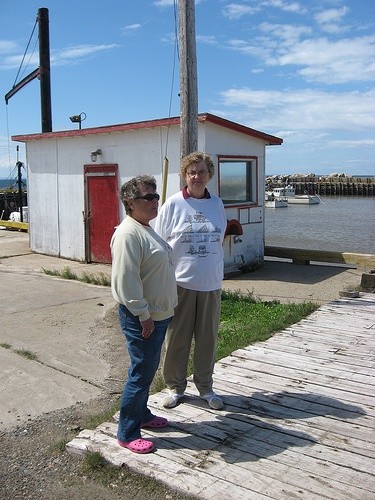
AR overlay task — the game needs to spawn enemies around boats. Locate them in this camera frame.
[265,184,323,204]
[264,192,289,208]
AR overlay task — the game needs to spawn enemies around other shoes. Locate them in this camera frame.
[199,392,225,410]
[161,390,184,409]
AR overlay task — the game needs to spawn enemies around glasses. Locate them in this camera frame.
[187,170,209,177]
[136,193,160,201]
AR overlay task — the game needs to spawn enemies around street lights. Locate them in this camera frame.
[69,112,87,129]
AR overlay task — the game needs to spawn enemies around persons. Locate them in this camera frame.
[110,175,177,454]
[154,151,227,409]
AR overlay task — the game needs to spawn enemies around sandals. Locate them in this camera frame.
[118,438,155,454]
[141,415,168,429]
